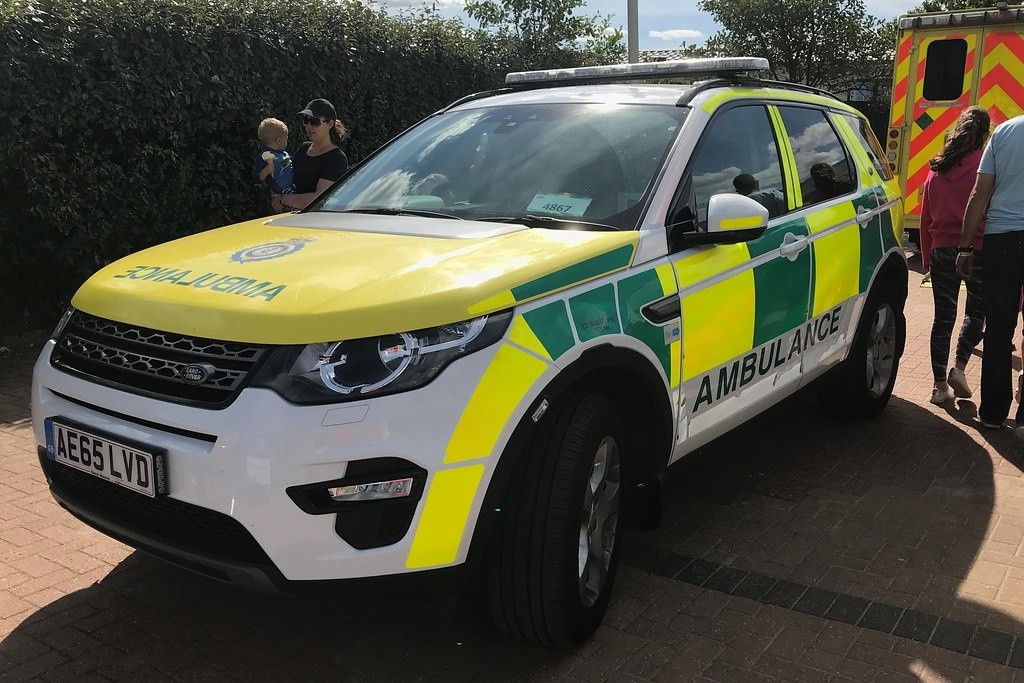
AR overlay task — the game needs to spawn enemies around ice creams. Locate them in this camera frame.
[261,151,276,177]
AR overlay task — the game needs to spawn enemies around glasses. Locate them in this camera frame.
[302,117,329,126]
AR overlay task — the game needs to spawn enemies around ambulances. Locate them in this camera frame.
[882,9,1024,253]
[29,59,907,658]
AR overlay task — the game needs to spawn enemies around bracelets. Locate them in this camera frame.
[280,194,284,206]
[958,252,973,257]
[958,247,973,252]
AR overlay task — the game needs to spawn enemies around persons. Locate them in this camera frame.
[732,174,785,218]
[919,107,997,404]
[271,98,348,214]
[955,115,1024,438]
[811,163,853,202]
[255,117,297,211]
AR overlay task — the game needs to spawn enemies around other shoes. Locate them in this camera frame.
[929,384,956,404]
[978,409,1001,429]
[1014,426,1024,443]
[947,366,973,399]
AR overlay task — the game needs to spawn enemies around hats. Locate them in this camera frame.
[296,98,338,121]
